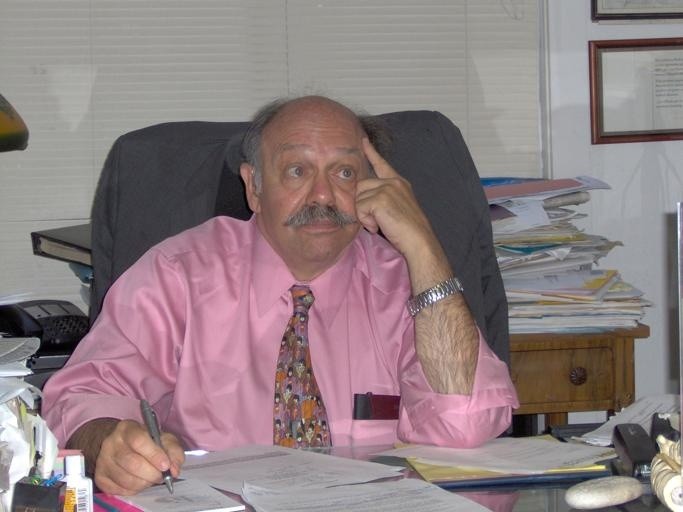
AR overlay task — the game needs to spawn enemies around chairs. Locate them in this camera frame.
[91,109,510,363]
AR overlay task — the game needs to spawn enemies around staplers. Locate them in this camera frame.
[612,423,660,494]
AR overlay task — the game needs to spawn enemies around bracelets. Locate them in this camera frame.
[406,277,463,319]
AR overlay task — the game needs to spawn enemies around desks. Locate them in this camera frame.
[509,323,649,428]
[94,444,672,512]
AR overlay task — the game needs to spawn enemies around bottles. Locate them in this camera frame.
[57,454,93,512]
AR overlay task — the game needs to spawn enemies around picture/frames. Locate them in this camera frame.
[591,0,683,20]
[588,37,683,145]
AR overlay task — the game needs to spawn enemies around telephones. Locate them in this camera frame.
[0,300,90,352]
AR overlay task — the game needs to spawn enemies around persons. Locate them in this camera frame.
[39,93,519,498]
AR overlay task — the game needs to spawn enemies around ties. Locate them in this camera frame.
[274,284,333,449]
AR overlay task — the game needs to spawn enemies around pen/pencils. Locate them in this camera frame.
[139,399,174,493]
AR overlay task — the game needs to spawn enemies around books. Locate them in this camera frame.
[479,176,652,333]
[69,262,93,284]
[31,224,91,267]
[0,337,42,406]
[92,479,246,512]
[393,433,610,489]
